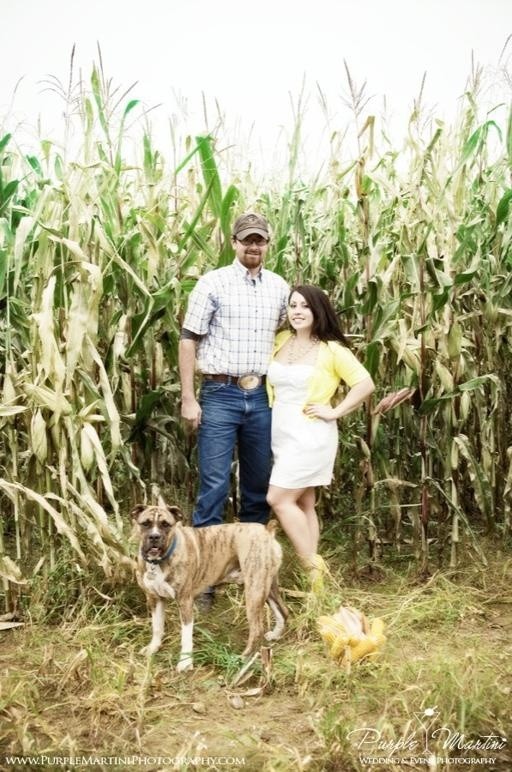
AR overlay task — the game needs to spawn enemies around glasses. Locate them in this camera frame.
[239,236,266,247]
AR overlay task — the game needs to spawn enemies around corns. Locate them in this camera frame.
[316,606,389,665]
[371,336,473,428]
[2,375,108,478]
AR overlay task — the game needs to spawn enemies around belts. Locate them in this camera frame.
[202,373,267,390]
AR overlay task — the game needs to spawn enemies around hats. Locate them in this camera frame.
[234,213,270,241]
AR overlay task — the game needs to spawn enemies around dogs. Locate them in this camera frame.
[128,503,284,674]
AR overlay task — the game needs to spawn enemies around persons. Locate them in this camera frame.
[266,284,375,588]
[177,214,293,601]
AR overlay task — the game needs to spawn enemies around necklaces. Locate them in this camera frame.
[288,335,318,366]
